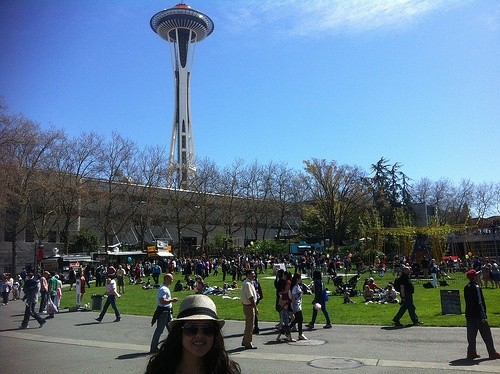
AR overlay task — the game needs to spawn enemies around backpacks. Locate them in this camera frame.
[394,278,401,292]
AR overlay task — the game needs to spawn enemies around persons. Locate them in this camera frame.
[0,272,22,306]
[392,266,423,326]
[145,294,241,374]
[273,269,306,343]
[94,274,121,322]
[68,266,75,291]
[464,269,500,360]
[19,271,63,329]
[393,253,472,279]
[126,249,352,284]
[430,259,439,288]
[363,277,395,304]
[224,281,238,288]
[116,265,126,294]
[80,263,116,288]
[174,275,194,292]
[472,256,500,289]
[242,269,263,349]
[145,279,154,287]
[150,273,178,354]
[374,256,387,277]
[304,271,332,328]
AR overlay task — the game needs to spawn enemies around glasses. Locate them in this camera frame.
[181,322,215,336]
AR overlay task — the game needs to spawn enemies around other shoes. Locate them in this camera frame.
[286,338,297,342]
[245,345,258,349]
[305,322,314,327]
[323,323,332,328]
[298,334,307,339]
[39,321,46,328]
[393,319,400,325]
[277,338,285,341]
[415,321,424,325]
[290,328,297,331]
[489,352,500,359]
[45,316,54,319]
[19,324,29,329]
[467,354,480,358]
[113,319,120,322]
[95,318,101,322]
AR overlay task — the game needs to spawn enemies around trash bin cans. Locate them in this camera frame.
[91,294,104,310]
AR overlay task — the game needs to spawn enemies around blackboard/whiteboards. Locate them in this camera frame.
[440,289,461,315]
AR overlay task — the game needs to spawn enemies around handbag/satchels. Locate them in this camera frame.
[47,298,57,315]
[324,293,328,301]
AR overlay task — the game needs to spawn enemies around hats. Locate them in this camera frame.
[466,269,481,279]
[167,294,225,332]
